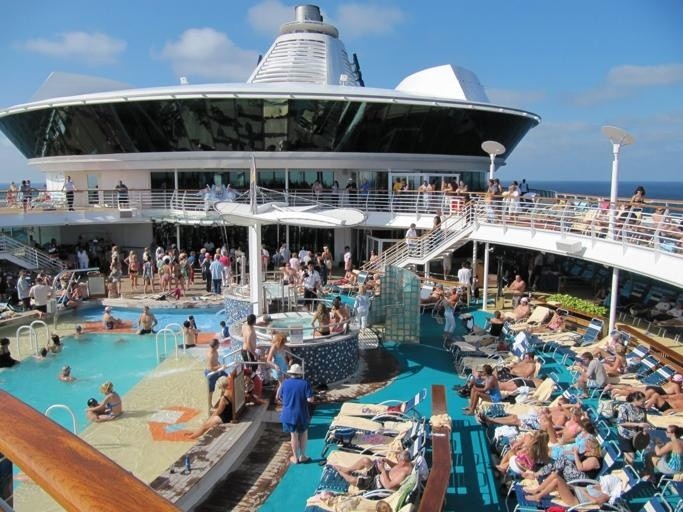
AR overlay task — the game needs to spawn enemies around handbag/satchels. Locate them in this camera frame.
[456,374,475,398]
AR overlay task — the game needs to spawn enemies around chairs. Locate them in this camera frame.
[407,277,470,318]
[446,299,683,512]
[304,387,428,512]
[331,268,370,296]
[529,198,683,254]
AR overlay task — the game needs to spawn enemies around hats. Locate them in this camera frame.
[104,307,112,311]
[286,364,305,375]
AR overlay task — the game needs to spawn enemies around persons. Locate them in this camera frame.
[1,177,683,512]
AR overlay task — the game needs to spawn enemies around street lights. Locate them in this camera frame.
[480,138,507,220]
[601,122,631,240]
